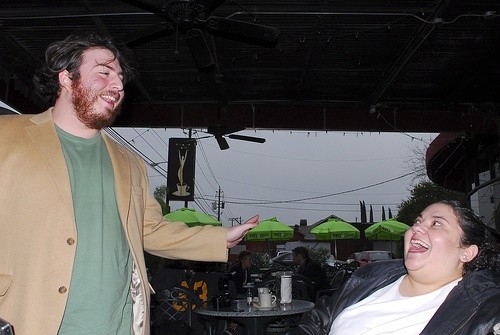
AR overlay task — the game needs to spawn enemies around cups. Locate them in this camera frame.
[210,297,222,310]
[279,275,292,304]
[258,287,272,302]
[260,294,277,306]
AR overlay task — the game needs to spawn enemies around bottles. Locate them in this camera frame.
[247,288,253,306]
[221,284,231,306]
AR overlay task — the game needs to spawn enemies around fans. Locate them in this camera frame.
[116,0,280,67]
[180,96,265,150]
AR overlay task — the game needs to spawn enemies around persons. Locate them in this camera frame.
[291,246,330,304]
[285,198,500,335]
[228,250,252,285]
[0,30,260,335]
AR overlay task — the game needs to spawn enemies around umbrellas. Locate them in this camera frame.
[161,207,413,259]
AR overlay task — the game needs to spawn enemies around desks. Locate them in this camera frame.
[190,300,314,335]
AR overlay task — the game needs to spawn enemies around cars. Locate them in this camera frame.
[261,250,293,265]
[324,251,401,274]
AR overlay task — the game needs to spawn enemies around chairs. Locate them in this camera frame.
[253,262,359,335]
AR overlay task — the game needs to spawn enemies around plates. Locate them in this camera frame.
[255,306,276,311]
